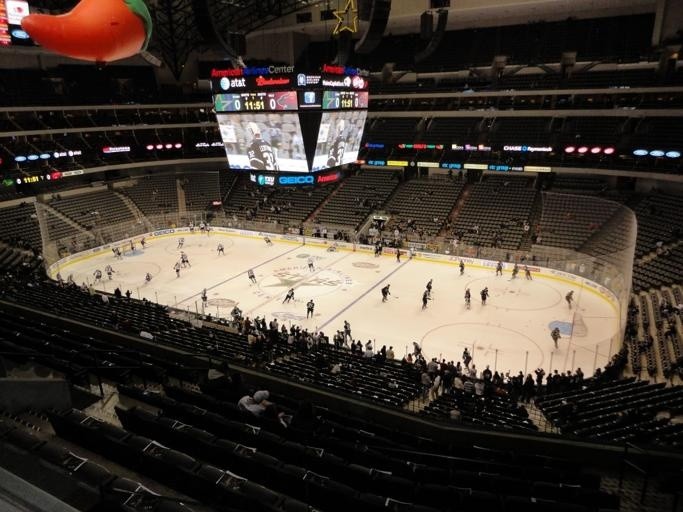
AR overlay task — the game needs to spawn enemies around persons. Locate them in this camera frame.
[326,119,344,167]
[380,283,391,302]
[179,251,191,268]
[109,309,120,330]
[199,288,209,309]
[215,242,224,255]
[188,221,193,231]
[199,221,204,234]
[55,265,131,305]
[280,287,294,304]
[140,237,145,248]
[463,287,471,309]
[176,238,184,248]
[479,285,490,305]
[449,405,461,422]
[307,260,315,272]
[311,214,432,262]
[129,239,134,251]
[112,247,123,261]
[425,278,433,292]
[245,121,280,171]
[206,223,210,235]
[263,235,271,246]
[229,303,682,404]
[565,290,575,310]
[173,260,181,278]
[144,271,152,281]
[420,289,431,310]
[197,360,235,402]
[445,216,542,282]
[236,389,287,423]
[237,181,312,235]
[306,299,314,319]
[247,268,256,283]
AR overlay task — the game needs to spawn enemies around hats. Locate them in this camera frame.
[254,390,270,403]
